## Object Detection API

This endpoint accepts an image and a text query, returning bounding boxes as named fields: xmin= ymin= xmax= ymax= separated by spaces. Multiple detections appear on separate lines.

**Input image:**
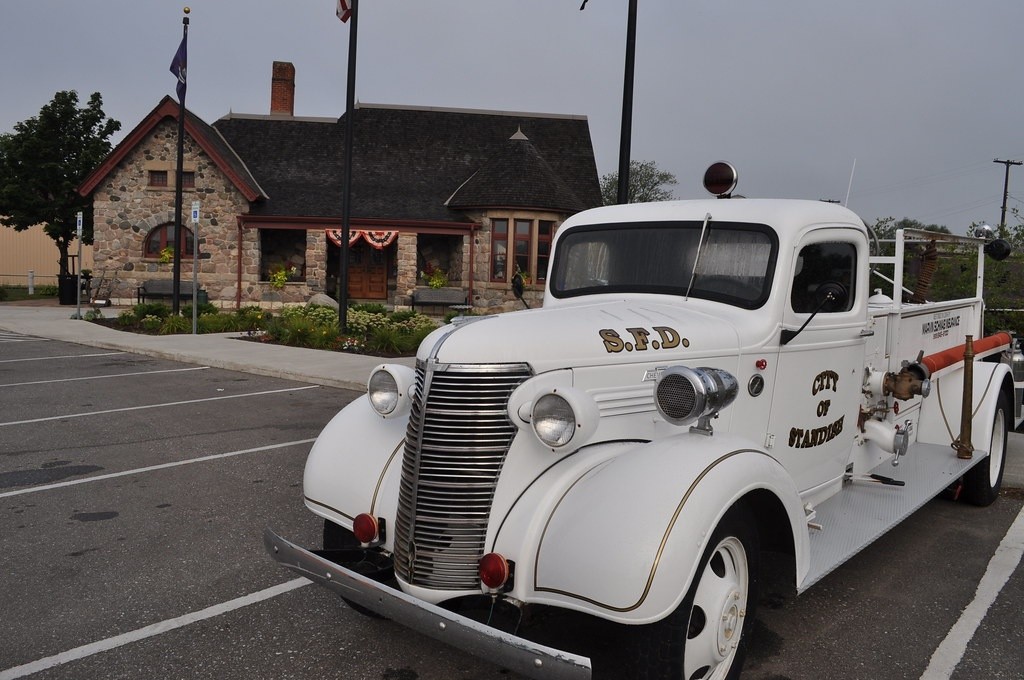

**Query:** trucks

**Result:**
xmin=263 ymin=158 xmax=1024 ymax=679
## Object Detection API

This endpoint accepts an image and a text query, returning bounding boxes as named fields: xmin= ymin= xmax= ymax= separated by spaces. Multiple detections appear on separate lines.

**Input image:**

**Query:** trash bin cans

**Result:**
xmin=56 ymin=274 xmax=79 ymax=305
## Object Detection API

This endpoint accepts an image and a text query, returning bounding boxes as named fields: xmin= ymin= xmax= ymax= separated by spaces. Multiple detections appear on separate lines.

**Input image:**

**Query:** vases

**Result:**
xmin=424 ymin=279 xmax=430 ymax=286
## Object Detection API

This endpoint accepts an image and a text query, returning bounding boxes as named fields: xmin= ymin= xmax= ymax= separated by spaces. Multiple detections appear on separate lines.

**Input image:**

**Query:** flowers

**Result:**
xmin=420 ymin=258 xmax=449 ymax=289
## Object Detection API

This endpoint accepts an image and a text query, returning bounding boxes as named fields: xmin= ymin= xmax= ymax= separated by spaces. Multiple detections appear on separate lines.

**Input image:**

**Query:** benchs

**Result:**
xmin=137 ymin=280 xmax=199 ymax=304
xmin=409 ymin=288 xmax=468 ymax=311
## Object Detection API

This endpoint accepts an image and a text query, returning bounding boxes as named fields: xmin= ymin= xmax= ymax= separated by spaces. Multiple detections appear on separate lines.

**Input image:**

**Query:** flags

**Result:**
xmin=169 ymin=26 xmax=187 ymax=107
xmin=336 ymin=0 xmax=351 ymax=23
